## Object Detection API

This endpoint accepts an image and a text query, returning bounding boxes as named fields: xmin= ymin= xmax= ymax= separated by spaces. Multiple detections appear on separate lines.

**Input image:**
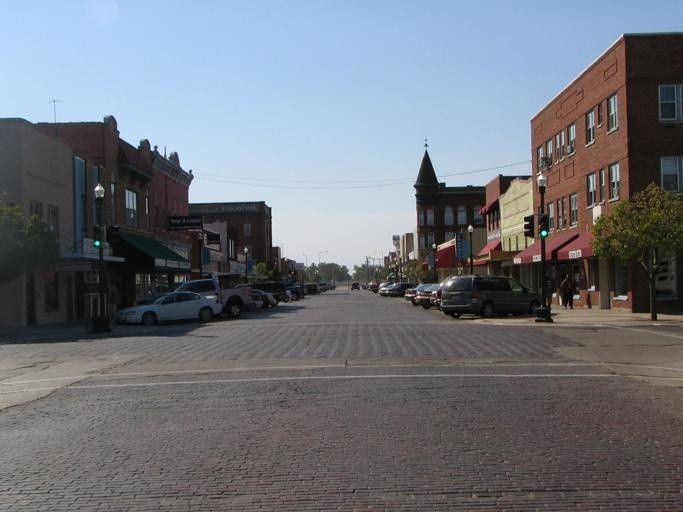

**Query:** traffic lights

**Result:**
xmin=93 ymin=225 xmax=120 ymax=249
xmin=524 ymin=214 xmax=549 ymax=238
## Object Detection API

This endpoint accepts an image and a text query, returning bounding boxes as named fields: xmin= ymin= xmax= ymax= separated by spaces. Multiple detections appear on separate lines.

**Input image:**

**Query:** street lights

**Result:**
xmin=292 ymin=272 xmax=293 ymax=276
xmin=198 ymin=233 xmax=202 ymax=279
xmin=376 ymin=268 xmax=380 ymax=281
xmin=244 ymin=247 xmax=248 ymax=282
xmin=535 ymin=174 xmax=553 ymax=321
xmin=389 ymin=255 xmax=402 ymax=284
xmin=94 ymin=184 xmax=105 ymax=317
xmin=284 ymin=257 xmax=288 ymax=280
xmin=468 ymin=224 xmax=473 ymax=275
xmin=433 ymin=243 xmax=436 ymax=283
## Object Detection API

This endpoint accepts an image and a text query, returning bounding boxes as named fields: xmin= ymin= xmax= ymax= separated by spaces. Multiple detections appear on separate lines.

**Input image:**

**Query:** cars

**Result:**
xmin=118 ymin=278 xmax=336 ymax=325
xmin=363 ymin=275 xmax=541 ymax=318
xmin=352 ymin=283 xmax=360 ymax=290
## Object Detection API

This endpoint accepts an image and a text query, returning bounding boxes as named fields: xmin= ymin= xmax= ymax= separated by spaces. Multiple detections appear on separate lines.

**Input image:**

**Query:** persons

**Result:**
xmin=540 ymin=272 xmax=554 ymax=307
xmin=559 ymin=272 xmax=576 ymax=308
